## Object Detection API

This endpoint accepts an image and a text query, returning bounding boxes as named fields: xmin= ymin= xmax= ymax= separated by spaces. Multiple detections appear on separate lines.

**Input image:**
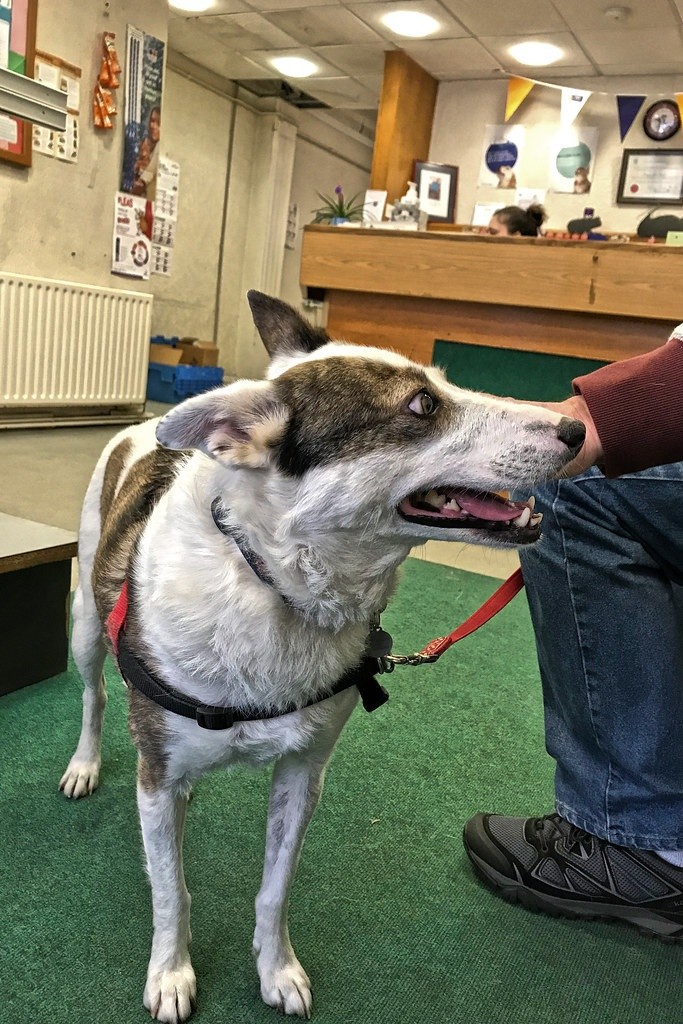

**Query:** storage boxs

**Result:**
xmin=0 ymin=270 xmax=153 ymax=410
xmin=175 ymin=336 xmax=219 ymax=367
xmin=148 ymin=363 xmax=224 ymax=404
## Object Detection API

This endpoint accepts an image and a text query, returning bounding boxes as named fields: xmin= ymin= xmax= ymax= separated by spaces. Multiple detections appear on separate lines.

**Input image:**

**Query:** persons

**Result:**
xmin=486 ymin=202 xmax=546 ymax=239
xmin=132 ymin=107 xmax=160 ymax=201
xmin=460 ymin=311 xmax=683 ymax=949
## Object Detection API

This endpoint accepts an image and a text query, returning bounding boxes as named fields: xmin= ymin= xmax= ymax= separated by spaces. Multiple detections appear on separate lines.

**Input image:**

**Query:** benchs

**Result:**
xmin=0 ymin=510 xmax=78 ymax=696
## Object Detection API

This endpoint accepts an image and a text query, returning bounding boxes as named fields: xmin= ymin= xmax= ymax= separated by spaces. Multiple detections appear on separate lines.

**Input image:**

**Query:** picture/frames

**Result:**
xmin=410 ymin=158 xmax=458 ymax=224
xmin=616 ymin=148 xmax=683 ymax=206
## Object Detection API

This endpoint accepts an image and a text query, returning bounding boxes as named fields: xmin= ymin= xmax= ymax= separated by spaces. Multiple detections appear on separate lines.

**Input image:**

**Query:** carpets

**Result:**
xmin=0 ymin=555 xmax=683 ymax=1024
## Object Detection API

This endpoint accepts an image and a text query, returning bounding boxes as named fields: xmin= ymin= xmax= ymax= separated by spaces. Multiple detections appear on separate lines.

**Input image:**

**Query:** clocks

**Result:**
xmin=642 ymin=99 xmax=681 ymax=141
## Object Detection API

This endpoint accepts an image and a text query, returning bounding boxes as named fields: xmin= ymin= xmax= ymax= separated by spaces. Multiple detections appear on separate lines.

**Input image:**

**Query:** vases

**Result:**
xmin=329 ymin=217 xmax=350 ymax=226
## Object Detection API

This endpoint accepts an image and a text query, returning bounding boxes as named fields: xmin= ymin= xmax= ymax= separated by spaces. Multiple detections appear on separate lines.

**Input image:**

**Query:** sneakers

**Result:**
xmin=463 ymin=812 xmax=683 ymax=941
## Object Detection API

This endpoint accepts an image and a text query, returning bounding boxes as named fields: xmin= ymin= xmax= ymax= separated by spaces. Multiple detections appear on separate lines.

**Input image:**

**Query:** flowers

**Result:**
xmin=299 ymin=185 xmax=377 ymax=230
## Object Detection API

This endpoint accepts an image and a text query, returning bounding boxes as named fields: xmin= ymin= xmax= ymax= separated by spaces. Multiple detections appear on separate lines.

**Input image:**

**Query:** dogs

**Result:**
xmin=57 ymin=287 xmax=589 ymax=1023
